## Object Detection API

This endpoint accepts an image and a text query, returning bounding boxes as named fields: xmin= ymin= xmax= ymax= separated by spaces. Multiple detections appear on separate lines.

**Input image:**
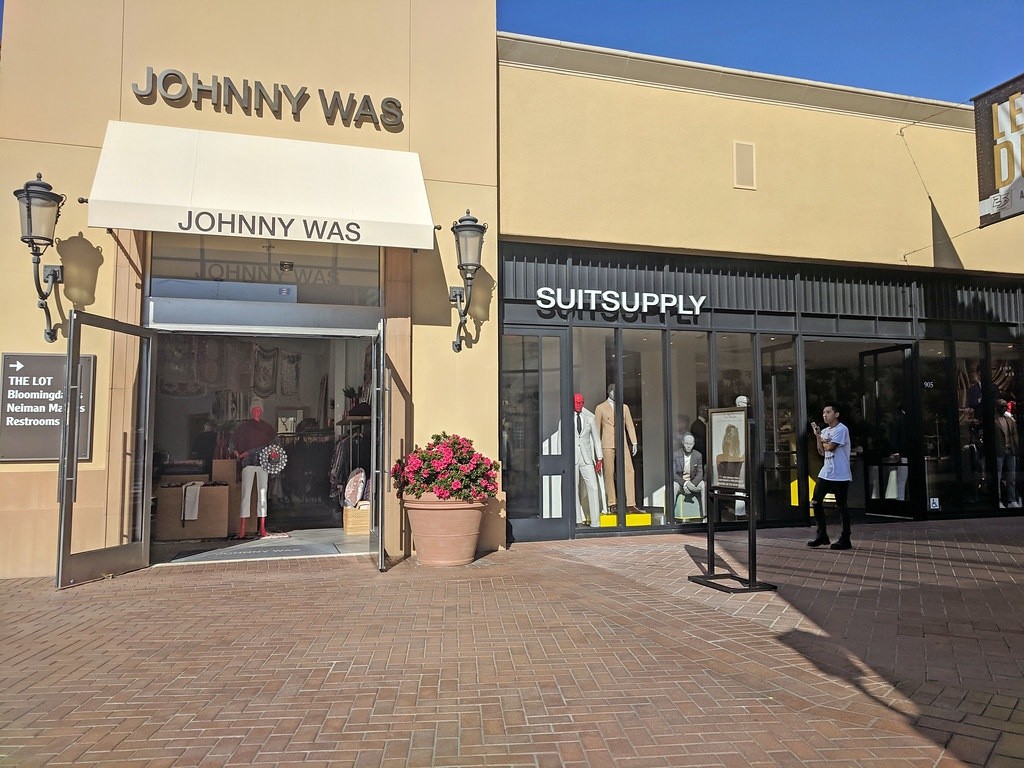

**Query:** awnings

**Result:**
xmin=86 ymin=119 xmax=434 ymax=250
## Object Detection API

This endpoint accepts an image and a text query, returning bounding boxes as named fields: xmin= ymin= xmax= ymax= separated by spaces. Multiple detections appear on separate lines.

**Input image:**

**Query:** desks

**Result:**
xmin=154 ymin=459 xmax=257 ymax=538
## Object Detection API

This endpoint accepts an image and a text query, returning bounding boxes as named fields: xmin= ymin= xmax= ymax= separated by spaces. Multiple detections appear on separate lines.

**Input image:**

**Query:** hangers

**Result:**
xmin=277 ymin=428 xmax=364 ymax=446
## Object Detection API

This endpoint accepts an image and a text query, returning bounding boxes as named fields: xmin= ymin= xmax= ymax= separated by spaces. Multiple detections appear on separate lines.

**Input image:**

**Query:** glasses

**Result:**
xmin=1000 ymin=405 xmax=1008 ymax=409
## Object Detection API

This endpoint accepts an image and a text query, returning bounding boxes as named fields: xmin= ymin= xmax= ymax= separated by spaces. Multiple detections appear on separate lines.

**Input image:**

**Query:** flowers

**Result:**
xmin=389 ymin=431 xmax=500 ymax=503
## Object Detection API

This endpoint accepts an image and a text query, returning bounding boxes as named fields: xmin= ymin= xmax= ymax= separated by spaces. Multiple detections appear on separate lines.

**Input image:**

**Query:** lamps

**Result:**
xmin=13 ymin=173 xmax=63 ymax=342
xmin=449 ymin=207 xmax=488 ymax=351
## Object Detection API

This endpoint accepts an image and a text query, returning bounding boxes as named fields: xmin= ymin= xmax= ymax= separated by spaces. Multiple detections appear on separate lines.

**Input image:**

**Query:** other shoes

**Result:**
xmin=703 ymin=518 xmax=707 ymax=523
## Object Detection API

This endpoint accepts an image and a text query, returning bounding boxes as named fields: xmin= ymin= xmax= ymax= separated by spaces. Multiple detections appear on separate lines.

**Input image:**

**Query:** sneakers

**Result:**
xmin=999 ymin=503 xmax=1006 ymax=508
xmin=807 ymin=533 xmax=830 ymax=547
xmin=1007 ymin=501 xmax=1022 ymax=508
xmin=830 ymin=538 xmax=852 ymax=549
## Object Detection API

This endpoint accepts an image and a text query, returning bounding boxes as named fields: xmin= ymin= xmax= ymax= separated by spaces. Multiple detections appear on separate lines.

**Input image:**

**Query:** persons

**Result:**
xmin=994 ymin=399 xmax=1020 ymax=508
xmin=191 ymin=418 xmax=218 ymax=462
xmin=594 ymin=383 xmax=646 ymax=513
xmin=228 ymin=396 xmax=276 ymax=537
xmin=671 ymin=431 xmax=708 ymax=523
xmin=556 ymin=392 xmax=604 ymax=527
xmin=805 ymin=405 xmax=853 ymax=550
xmin=735 ymin=395 xmax=750 ymax=409
xmin=690 ymin=404 xmax=709 ymax=476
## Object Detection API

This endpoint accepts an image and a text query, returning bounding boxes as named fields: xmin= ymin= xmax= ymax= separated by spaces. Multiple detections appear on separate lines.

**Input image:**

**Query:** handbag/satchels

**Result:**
xmin=278 ymin=417 xmax=296 ymax=433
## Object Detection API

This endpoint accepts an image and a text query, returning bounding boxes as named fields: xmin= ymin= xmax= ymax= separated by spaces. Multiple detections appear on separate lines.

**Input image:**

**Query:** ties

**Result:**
xmin=576 ymin=413 xmax=582 ymax=435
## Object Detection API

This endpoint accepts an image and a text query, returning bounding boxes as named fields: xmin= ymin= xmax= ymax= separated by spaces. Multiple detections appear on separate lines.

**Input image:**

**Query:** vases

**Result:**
xmin=401 ymin=491 xmax=488 ymax=566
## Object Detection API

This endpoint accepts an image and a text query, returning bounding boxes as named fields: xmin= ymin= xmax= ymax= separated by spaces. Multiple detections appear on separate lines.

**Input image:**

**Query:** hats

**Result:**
xmin=250 ymin=396 xmax=263 ymax=413
xmin=608 ymin=384 xmax=615 ymax=394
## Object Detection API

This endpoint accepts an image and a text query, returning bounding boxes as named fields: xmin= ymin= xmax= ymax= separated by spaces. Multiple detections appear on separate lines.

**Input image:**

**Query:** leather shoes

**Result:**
xmin=627 ymin=506 xmax=646 ymax=514
xmin=611 ymin=505 xmax=618 ymax=512
